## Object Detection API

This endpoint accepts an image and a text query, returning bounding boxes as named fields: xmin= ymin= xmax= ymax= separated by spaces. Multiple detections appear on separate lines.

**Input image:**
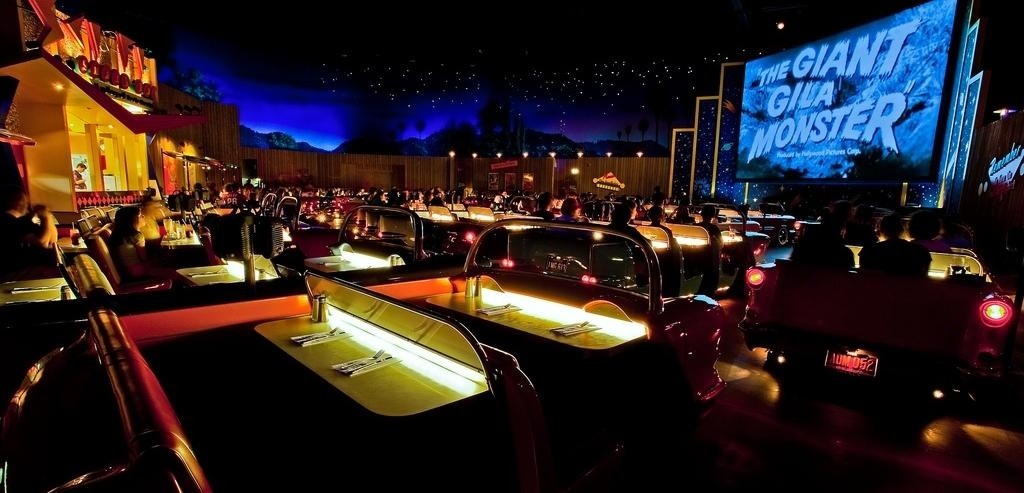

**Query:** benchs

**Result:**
xmin=1 ymin=184 xmax=1023 ymax=490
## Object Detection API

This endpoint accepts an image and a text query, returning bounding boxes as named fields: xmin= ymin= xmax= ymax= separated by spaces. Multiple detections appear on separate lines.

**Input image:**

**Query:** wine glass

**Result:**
xmin=163 ymin=219 xmax=181 ymax=239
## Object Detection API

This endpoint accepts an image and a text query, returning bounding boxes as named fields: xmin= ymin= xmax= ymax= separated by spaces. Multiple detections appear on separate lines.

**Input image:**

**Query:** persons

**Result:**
xmin=73 ymin=163 xmax=87 ymax=189
xmin=1 ymin=172 xmax=58 ymax=281
xmin=108 ymin=179 xmax=953 ymax=280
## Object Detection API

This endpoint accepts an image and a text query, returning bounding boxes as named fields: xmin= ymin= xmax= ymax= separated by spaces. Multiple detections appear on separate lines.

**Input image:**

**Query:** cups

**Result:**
xmin=175 ymin=225 xmax=184 ymax=240
xmin=70 ymin=229 xmax=80 ymax=246
xmin=185 ymin=224 xmax=194 ymax=239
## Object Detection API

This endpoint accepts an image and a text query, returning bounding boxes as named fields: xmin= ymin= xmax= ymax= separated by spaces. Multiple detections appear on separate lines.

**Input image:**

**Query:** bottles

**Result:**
xmin=474 ymin=274 xmax=482 ymax=297
xmin=312 ymin=294 xmax=328 ymax=323
xmin=464 ymin=275 xmax=473 ymax=299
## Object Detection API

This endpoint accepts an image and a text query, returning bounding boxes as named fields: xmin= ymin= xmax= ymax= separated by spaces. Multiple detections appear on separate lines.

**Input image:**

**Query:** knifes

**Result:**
xmin=343 ymin=356 xmax=395 ymax=379
xmin=297 ymin=331 xmax=346 ymax=346
xmin=559 ymin=327 xmax=604 ymax=337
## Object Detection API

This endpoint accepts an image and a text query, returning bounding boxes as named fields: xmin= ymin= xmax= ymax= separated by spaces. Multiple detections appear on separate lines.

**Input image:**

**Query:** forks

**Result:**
xmin=552 ymin=320 xmax=589 ymax=333
xmin=477 ymin=303 xmax=511 ymax=314
xmin=292 ymin=326 xmax=341 ymax=342
xmin=13 ymin=281 xmax=63 ymax=290
xmin=340 ymin=348 xmax=385 ymax=370
